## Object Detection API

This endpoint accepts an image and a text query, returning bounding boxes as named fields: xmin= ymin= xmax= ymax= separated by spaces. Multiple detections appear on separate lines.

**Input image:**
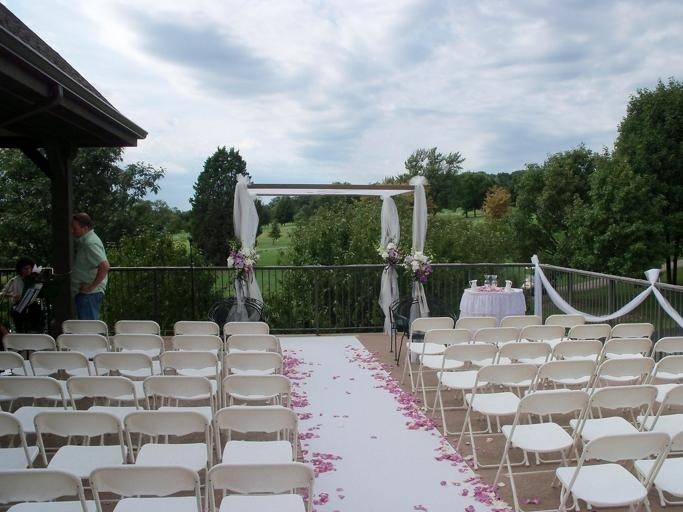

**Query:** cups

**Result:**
xmin=482 ymin=274 xmax=497 ymax=289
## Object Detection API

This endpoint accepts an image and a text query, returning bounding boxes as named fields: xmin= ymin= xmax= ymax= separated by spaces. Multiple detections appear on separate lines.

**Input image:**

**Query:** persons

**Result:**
xmin=66 ymin=211 xmax=112 ymax=320
xmin=0 ymin=256 xmax=47 ymax=334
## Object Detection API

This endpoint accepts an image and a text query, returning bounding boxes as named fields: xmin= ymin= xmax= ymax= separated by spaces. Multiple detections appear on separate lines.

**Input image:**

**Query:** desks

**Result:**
xmin=458 ymin=286 xmax=526 ymax=325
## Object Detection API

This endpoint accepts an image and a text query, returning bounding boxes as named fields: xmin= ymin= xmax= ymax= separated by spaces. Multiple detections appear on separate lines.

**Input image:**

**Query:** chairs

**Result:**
xmin=140 ymin=376 xmax=215 ymax=453
xmin=90 ymin=464 xmax=203 ymax=512
xmin=158 ymin=351 xmax=219 ymax=408
xmin=406 ymin=316 xmax=454 ymax=396
xmin=174 ymin=320 xmax=220 ymax=336
xmin=62 ymin=319 xmax=108 ymax=335
xmin=0 ymin=351 xmax=32 ymax=411
xmin=222 ymin=352 xmax=283 ymax=405
xmin=555 ymin=431 xmax=670 ymax=510
xmin=429 ymin=343 xmax=494 ymax=436
xmin=454 ymin=314 xmax=682 ymax=365
xmin=28 ymin=351 xmax=92 ymax=408
xmin=66 ymin=375 xmax=143 ymax=449
xmin=93 ymin=352 xmax=155 ymax=408
xmin=413 ymin=329 xmax=470 ymax=412
xmin=223 ymin=322 xmax=270 ymax=334
xmin=171 ymin=335 xmax=224 ymax=376
xmin=634 ymin=431 xmax=681 ymax=510
xmin=489 ymin=387 xmax=590 ymax=512
xmin=521 ymin=359 xmax=596 ymax=465
xmin=34 ymin=409 xmax=127 ymax=503
xmin=56 ymin=334 xmax=111 ymax=376
xmin=112 ymin=333 xmax=164 ymax=373
xmin=585 ymin=357 xmax=654 ymax=423
xmin=0 ymin=410 xmax=38 ymax=468
xmin=637 ymin=383 xmax=683 ymax=451
xmin=0 ymin=374 xmax=75 ymax=448
xmin=216 ymin=405 xmax=299 ymax=497
xmin=221 ymin=374 xmax=291 ymax=441
xmin=457 ymin=363 xmax=538 ymax=471
xmin=651 ymin=354 xmax=681 ymax=385
xmin=123 ymin=410 xmax=213 ymax=511
xmin=226 ymin=334 xmax=283 ymax=376
xmin=114 ymin=319 xmax=161 ymax=335
xmin=206 ymin=463 xmax=312 ymax=512
xmin=0 ymin=467 xmax=89 ymax=512
xmin=3 ymin=333 xmax=56 ymax=374
xmin=556 ymin=384 xmax=659 ymax=488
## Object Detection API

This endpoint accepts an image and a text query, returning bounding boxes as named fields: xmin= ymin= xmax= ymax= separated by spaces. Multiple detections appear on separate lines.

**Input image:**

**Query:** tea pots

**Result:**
xmin=505 ymin=280 xmax=512 ymax=291
xmin=468 ymin=279 xmax=478 ymax=292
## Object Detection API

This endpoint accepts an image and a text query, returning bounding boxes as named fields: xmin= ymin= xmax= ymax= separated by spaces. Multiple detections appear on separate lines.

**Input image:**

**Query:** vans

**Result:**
xmin=520 ymin=275 xmax=556 ymax=295
